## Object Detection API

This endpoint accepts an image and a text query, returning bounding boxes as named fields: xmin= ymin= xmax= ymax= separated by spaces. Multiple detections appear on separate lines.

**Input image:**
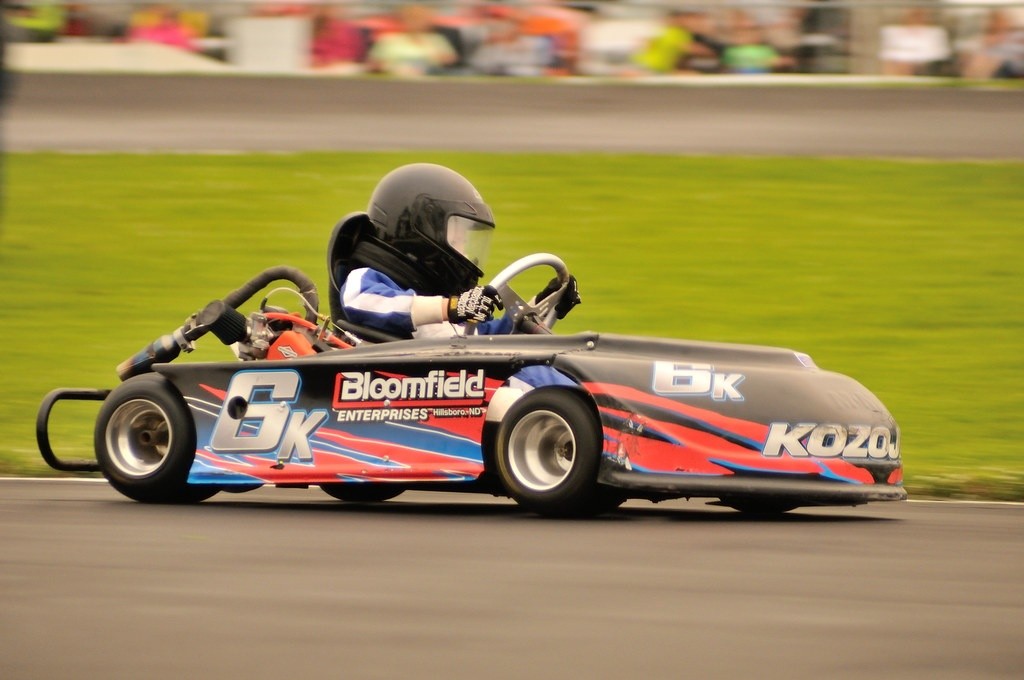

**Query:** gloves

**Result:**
xmin=447 ymin=285 xmax=503 ymax=324
xmin=535 ymin=274 xmax=581 ymax=320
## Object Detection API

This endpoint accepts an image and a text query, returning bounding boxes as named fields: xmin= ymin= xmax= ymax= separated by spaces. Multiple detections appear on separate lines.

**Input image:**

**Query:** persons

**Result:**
xmin=340 ymin=163 xmax=584 ymax=342
xmin=3 ymin=1 xmax=1024 ymax=83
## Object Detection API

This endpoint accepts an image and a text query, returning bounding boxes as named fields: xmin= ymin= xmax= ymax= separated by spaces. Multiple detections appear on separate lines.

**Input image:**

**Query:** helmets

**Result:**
xmin=367 ymin=163 xmax=496 ymax=298
